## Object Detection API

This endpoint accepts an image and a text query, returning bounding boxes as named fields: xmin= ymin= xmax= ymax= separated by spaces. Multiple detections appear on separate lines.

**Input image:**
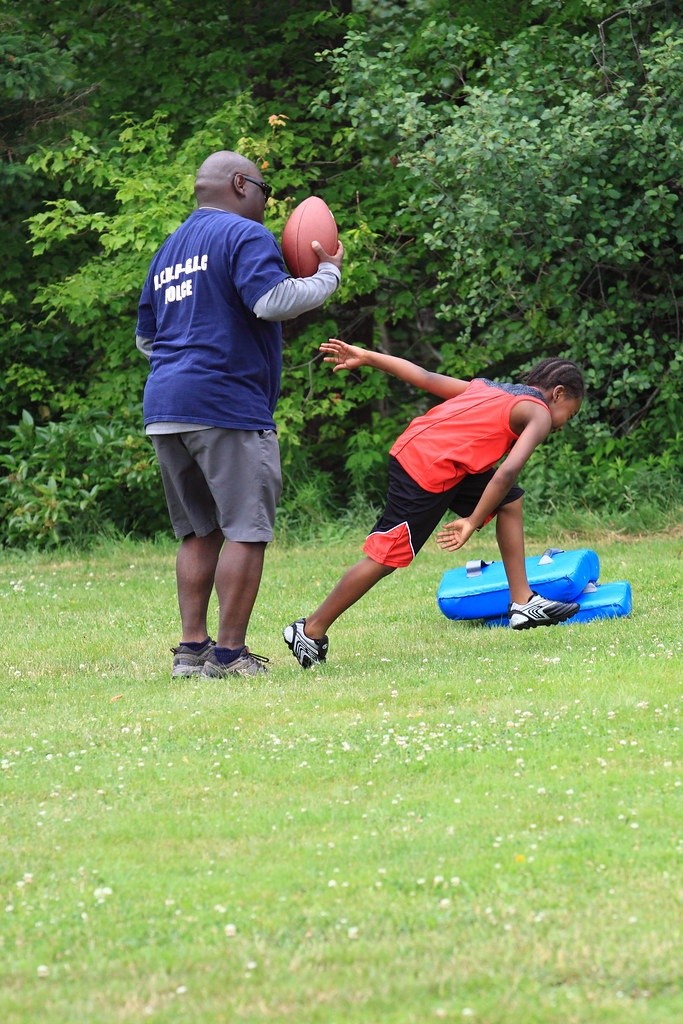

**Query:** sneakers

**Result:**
xmin=283 ymin=618 xmax=329 ymax=670
xmin=508 ymin=590 xmax=580 ymax=630
xmin=170 ymin=637 xmax=216 ymax=679
xmin=201 ymin=646 xmax=269 ymax=681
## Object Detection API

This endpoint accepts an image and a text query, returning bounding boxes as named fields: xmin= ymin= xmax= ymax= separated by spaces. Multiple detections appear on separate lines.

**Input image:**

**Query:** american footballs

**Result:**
xmin=282 ymin=196 xmax=338 ymax=279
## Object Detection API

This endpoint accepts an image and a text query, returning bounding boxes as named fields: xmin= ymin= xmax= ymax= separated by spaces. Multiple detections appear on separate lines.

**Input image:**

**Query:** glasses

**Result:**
xmin=235 ymin=173 xmax=272 ymax=204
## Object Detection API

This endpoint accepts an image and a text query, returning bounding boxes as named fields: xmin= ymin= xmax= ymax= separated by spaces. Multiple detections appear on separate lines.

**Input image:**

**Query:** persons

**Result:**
xmin=281 ymin=336 xmax=583 ymax=668
xmin=133 ymin=149 xmax=344 ymax=685
